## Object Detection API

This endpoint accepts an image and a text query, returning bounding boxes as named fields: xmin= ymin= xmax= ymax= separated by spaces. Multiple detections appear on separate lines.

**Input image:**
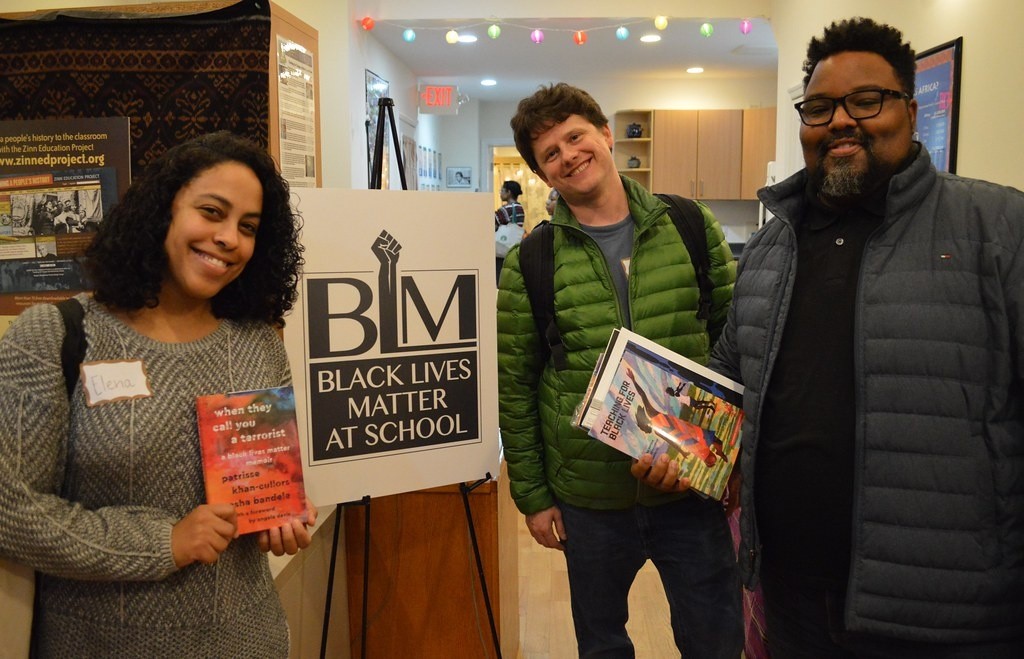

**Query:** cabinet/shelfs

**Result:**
xmin=612 ymin=109 xmax=777 ymax=200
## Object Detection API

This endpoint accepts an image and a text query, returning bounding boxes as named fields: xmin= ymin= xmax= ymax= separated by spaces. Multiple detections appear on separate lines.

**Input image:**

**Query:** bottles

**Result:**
xmin=627 ymin=156 xmax=640 ymax=168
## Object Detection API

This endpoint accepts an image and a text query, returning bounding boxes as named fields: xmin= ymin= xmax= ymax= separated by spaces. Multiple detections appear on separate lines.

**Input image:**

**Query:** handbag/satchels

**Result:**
xmin=495 ymin=202 xmax=527 ymax=259
xmin=0 ymin=298 xmax=89 ymax=659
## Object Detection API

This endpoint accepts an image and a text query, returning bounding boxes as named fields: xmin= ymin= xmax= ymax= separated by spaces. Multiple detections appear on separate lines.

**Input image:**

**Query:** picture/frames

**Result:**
xmin=365 ymin=69 xmax=390 ymax=190
xmin=446 ymin=167 xmax=472 ymax=188
xmin=915 ymin=36 xmax=964 ymax=177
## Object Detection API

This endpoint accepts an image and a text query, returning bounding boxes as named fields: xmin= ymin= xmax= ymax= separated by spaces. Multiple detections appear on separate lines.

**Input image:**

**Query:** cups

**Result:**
xmin=627 ymin=124 xmax=643 ymax=138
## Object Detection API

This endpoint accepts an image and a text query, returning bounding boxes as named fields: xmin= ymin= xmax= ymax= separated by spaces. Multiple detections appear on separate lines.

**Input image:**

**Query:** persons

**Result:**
xmin=452 ymin=172 xmax=470 ymax=185
xmin=630 ymin=16 xmax=1024 ymax=659
xmin=545 ymin=187 xmax=560 ymax=215
xmin=0 ymin=129 xmax=318 ymax=659
xmin=497 ymin=84 xmax=744 ymax=659
xmin=495 ymin=180 xmax=525 ymax=289
xmin=34 ymin=193 xmax=88 ymax=235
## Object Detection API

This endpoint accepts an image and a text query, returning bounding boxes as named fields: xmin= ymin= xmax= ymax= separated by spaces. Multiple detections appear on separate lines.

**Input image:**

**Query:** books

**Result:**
xmin=194 ymin=385 xmax=309 ymax=540
xmin=571 ymin=324 xmax=746 ymax=500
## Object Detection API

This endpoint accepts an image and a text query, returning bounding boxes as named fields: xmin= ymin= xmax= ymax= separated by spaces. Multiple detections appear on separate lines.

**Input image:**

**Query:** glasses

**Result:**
xmin=794 ymin=86 xmax=910 ymax=127
